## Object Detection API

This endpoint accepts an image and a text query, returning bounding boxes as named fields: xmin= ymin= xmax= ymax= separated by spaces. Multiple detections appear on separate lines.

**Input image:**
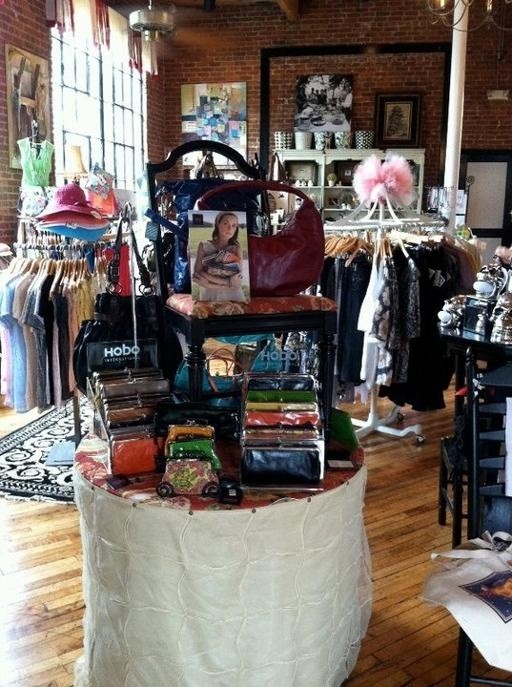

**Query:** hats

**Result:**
xmin=33 ymin=174 xmax=112 ymax=242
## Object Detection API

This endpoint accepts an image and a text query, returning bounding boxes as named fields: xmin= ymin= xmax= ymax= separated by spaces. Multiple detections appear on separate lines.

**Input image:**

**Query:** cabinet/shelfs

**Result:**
xmin=275 ymin=146 xmax=423 ymax=224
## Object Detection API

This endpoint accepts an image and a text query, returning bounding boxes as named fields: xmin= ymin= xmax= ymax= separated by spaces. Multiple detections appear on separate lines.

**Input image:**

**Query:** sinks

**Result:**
xmin=449 ymin=295 xmax=499 ymax=335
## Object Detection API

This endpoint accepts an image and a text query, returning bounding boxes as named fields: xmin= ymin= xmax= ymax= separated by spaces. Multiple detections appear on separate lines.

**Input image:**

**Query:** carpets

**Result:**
xmin=0 ymin=395 xmax=90 ymax=502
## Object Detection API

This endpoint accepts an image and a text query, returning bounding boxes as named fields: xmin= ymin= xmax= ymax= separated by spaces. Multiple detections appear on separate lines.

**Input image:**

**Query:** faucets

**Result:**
xmin=480 ymin=263 xmax=509 ymax=301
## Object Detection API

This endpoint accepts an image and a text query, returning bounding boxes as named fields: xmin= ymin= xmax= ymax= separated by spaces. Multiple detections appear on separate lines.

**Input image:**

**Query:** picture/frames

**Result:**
xmin=373 ymin=89 xmax=424 ymax=147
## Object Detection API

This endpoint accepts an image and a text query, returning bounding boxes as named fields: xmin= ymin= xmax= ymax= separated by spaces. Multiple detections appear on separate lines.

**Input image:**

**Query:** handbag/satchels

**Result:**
xmin=415 ymin=529 xmax=512 ymax=672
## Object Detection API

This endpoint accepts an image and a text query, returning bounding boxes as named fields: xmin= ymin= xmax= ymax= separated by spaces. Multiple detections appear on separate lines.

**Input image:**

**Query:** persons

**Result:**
xmin=307 ymin=87 xmax=327 ymax=106
xmin=192 ymin=211 xmax=248 ymax=300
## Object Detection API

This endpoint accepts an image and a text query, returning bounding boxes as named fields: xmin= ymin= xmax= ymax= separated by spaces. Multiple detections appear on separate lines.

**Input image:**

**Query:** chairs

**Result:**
xmin=436 ymin=342 xmax=512 ymax=687
xmin=145 ymin=137 xmax=338 ymax=430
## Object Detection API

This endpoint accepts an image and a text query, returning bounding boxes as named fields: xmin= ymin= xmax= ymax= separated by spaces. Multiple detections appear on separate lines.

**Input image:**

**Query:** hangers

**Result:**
xmin=323 ymin=231 xmax=484 ymax=269
xmin=5 ymin=242 xmax=110 ymax=280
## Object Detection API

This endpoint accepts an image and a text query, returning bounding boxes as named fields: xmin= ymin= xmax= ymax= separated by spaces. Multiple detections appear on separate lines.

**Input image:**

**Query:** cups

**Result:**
xmin=274 ymin=129 xmax=377 ymax=150
